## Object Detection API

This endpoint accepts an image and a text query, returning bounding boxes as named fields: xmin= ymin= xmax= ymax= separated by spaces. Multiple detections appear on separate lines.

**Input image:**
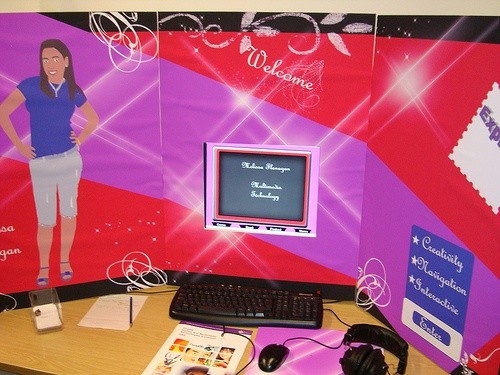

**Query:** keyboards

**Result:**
xmin=169 ymin=284 xmax=322 ymax=329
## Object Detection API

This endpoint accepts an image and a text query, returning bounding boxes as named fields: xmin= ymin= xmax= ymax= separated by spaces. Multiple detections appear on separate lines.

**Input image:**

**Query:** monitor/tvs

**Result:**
xmin=202 ymin=142 xmax=320 ymax=238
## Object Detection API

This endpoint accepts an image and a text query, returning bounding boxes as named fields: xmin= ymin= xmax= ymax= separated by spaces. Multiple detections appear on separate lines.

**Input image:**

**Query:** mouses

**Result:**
xmin=258 ymin=344 xmax=289 ymax=373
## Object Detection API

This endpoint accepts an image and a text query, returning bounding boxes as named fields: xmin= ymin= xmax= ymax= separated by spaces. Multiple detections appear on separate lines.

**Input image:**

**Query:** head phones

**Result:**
xmin=340 ymin=324 xmax=409 ymax=375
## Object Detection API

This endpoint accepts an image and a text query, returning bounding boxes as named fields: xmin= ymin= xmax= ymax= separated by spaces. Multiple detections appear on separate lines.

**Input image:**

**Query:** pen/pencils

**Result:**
xmin=130 ymin=296 xmax=132 ymax=327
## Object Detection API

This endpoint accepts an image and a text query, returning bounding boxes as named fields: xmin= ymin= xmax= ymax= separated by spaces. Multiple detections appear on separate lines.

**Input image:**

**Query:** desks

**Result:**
xmin=0 ymin=284 xmax=453 ymax=375
